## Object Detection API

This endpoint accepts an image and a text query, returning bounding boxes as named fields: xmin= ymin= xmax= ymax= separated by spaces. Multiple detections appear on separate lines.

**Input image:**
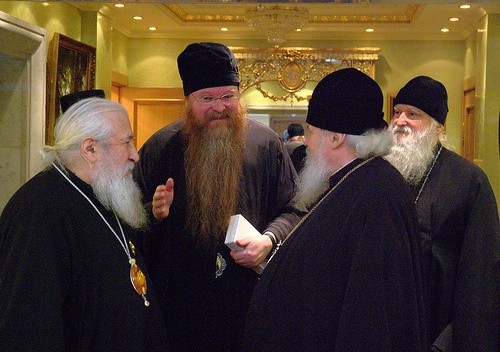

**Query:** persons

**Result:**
xmin=132 ymin=42 xmax=302 ymax=352
xmin=0 ymin=98 xmax=167 ymax=352
xmin=241 ymin=68 xmax=428 ymax=352
xmin=382 ymin=76 xmax=500 ymax=352
xmin=286 ymin=124 xmax=307 ymax=175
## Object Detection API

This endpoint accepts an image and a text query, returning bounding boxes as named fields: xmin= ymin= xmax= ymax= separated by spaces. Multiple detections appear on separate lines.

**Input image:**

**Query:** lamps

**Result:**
xmin=244 ymin=2 xmax=310 ymax=49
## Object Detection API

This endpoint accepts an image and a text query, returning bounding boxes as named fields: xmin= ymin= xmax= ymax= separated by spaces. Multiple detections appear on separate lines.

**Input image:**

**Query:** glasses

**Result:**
xmin=191 ymin=93 xmax=239 ymax=105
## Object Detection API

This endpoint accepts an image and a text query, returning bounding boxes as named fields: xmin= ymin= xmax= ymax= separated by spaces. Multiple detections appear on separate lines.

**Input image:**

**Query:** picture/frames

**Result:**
xmin=386 ymin=91 xmax=397 ymax=124
xmin=45 ymin=31 xmax=97 ymax=148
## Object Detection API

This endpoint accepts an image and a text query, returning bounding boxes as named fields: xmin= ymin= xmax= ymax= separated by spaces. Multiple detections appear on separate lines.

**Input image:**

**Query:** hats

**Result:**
xmin=177 ymin=41 xmax=240 ymax=97
xmin=394 ymin=75 xmax=449 ymax=126
xmin=287 ymin=124 xmax=304 ymax=139
xmin=306 ymin=67 xmax=389 ymax=136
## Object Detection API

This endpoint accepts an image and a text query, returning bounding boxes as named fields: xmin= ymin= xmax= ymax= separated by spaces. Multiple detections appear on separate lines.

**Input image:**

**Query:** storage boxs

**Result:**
xmin=224 ymin=214 xmax=269 ymax=277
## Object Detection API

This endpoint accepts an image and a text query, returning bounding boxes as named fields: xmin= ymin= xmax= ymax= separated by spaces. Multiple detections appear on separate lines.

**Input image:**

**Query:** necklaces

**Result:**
xmin=267 ymin=155 xmax=377 ymax=264
xmin=415 ymin=145 xmax=443 ymax=202
xmin=51 ymin=160 xmax=149 ymax=306
